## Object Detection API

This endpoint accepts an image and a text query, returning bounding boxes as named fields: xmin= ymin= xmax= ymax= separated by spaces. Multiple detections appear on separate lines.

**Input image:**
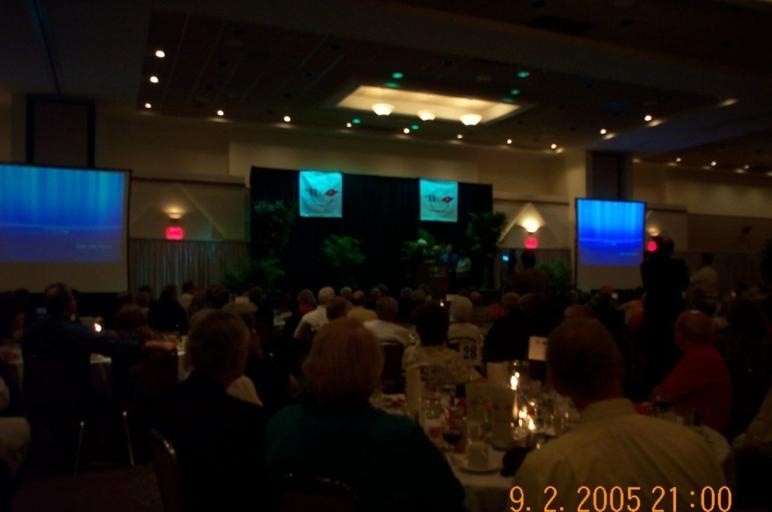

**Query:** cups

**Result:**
xmin=399 ymin=361 xmax=577 ymax=462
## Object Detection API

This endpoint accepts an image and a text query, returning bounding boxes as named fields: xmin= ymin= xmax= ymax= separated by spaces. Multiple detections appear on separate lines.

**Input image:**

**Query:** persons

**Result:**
xmin=1 ymin=235 xmax=772 ymax=510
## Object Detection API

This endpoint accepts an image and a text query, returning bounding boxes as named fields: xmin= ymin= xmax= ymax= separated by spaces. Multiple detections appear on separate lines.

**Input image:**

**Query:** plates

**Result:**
xmin=460 ymin=460 xmax=499 ymax=475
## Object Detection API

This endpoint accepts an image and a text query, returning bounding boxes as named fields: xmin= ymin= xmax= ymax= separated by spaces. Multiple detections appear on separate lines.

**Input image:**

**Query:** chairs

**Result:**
xmin=377 ymin=338 xmax=405 ymax=392
xmin=447 ymin=336 xmax=477 ymax=356
